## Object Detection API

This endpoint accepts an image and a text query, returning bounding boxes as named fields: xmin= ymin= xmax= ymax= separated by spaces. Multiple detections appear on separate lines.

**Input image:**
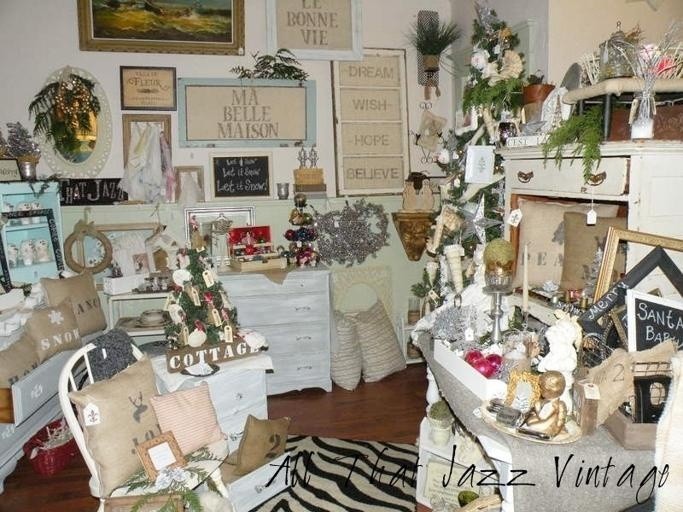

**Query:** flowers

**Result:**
xmin=463 ymin=2 xmax=525 ymax=110
xmin=7 ymin=122 xmax=41 ymax=162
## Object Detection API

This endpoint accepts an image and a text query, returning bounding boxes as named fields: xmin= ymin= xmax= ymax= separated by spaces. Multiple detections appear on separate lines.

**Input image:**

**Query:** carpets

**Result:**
xmin=249 ymin=433 xmax=420 ymax=512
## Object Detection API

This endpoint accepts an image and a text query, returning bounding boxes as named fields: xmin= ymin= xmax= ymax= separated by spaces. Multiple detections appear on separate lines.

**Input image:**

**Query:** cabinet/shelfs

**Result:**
xmin=214 ymin=259 xmax=335 ymax=396
xmin=492 ymin=137 xmax=682 ymax=333
xmin=0 ymin=329 xmax=106 ymax=494
xmin=150 ymin=353 xmax=274 ymax=435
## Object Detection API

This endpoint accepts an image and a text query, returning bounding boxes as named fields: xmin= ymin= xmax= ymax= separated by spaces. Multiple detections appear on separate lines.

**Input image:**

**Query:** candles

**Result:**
xmin=522 ymin=244 xmax=528 ymax=311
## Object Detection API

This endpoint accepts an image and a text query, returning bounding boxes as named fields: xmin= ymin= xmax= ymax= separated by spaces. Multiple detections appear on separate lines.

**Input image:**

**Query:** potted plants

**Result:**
xmin=406 ymin=335 xmax=422 ymax=359
xmin=523 ymin=74 xmax=556 ymax=102
xmin=483 ymin=238 xmax=515 ymax=291
xmin=402 ymin=19 xmax=462 ymax=70
xmin=406 ymin=297 xmax=419 ymax=324
xmin=425 ymin=401 xmax=454 ymax=448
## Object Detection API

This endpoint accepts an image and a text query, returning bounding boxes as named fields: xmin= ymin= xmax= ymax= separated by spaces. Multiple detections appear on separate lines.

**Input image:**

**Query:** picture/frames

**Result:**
xmin=119 ymin=66 xmax=177 ymax=113
xmin=266 ymin=1 xmax=363 ymax=63
xmin=0 ymin=209 xmax=65 ymax=291
xmin=135 ymin=430 xmax=184 ymax=481
xmin=184 ymin=205 xmax=257 ymax=269
xmin=79 ymin=224 xmax=165 ymax=287
xmin=0 ymin=181 xmax=65 ymax=283
xmin=170 ymin=164 xmax=205 ymax=202
xmin=76 ymin=0 xmax=247 ymax=58
xmin=330 ymin=45 xmax=410 ymax=196
xmin=575 ymin=245 xmax=682 ymax=348
xmin=0 ymin=158 xmax=22 ymax=180
xmin=175 ymin=76 xmax=316 ymax=149
xmin=35 ymin=64 xmax=112 ymax=180
xmin=121 ymin=114 xmax=171 ymax=170
xmin=594 ymin=226 xmax=683 ymax=306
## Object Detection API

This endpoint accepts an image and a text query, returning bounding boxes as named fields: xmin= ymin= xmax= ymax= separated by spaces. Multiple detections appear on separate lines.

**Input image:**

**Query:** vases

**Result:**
xmin=629 ymin=89 xmax=655 ymax=140
xmin=475 ymin=99 xmax=510 ymax=145
xmin=16 ymin=160 xmax=36 ymax=180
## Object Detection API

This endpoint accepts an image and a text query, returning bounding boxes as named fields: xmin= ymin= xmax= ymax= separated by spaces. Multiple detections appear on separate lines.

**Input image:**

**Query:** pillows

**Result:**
xmin=233 ymin=414 xmax=291 ymax=477
xmin=66 ymin=351 xmax=163 ymax=495
xmin=103 ymin=493 xmax=184 ymax=512
xmin=28 ymin=296 xmax=82 ymax=358
xmin=329 ymin=309 xmax=361 ymax=392
xmin=351 ymin=301 xmax=407 ymax=383
xmin=146 ymin=380 xmax=227 ymax=456
xmin=39 ymin=275 xmax=107 ymax=336
xmin=0 ymin=333 xmax=40 ymax=391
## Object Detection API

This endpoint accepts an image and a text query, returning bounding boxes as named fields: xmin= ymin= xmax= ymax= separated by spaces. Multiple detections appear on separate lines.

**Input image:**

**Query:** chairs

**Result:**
xmin=59 ymin=333 xmax=234 ymax=512
